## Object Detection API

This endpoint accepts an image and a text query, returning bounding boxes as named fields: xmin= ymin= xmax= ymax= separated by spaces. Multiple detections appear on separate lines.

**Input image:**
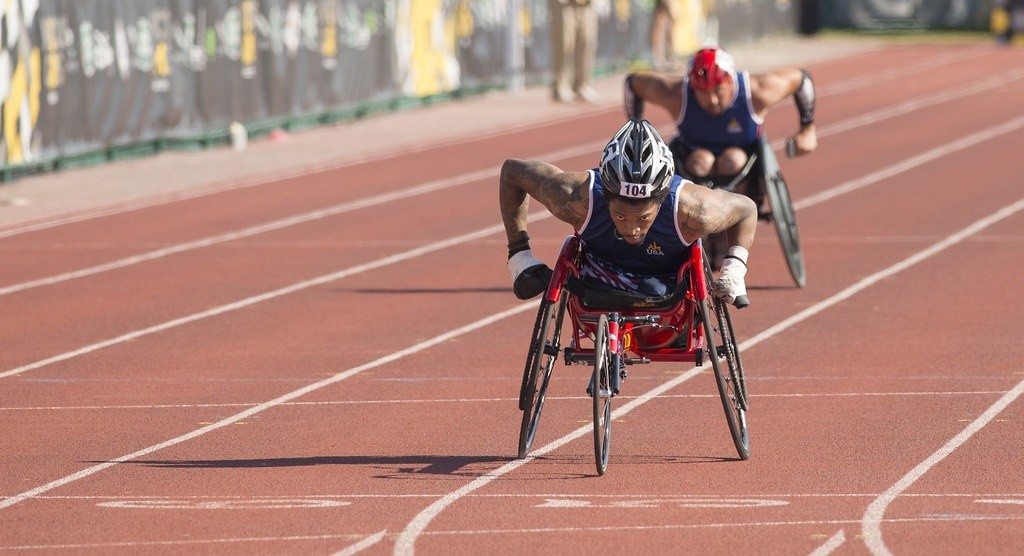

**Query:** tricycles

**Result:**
xmin=667 ymin=132 xmax=807 ymax=289
xmin=514 ymin=233 xmax=752 ymax=476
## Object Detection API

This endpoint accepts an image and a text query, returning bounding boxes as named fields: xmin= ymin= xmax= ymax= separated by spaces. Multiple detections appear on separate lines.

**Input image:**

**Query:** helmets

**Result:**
xmin=599 ymin=119 xmax=675 ymax=198
xmin=688 ymin=47 xmax=735 ymax=89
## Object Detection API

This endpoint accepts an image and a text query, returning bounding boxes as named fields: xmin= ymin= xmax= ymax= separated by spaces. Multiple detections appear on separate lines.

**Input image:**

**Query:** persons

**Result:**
xmin=546 ymin=0 xmax=600 ymax=110
xmin=622 ymin=46 xmax=819 ymax=271
xmin=649 ymin=0 xmax=682 ymax=70
xmin=500 ymin=119 xmax=758 ymax=351
